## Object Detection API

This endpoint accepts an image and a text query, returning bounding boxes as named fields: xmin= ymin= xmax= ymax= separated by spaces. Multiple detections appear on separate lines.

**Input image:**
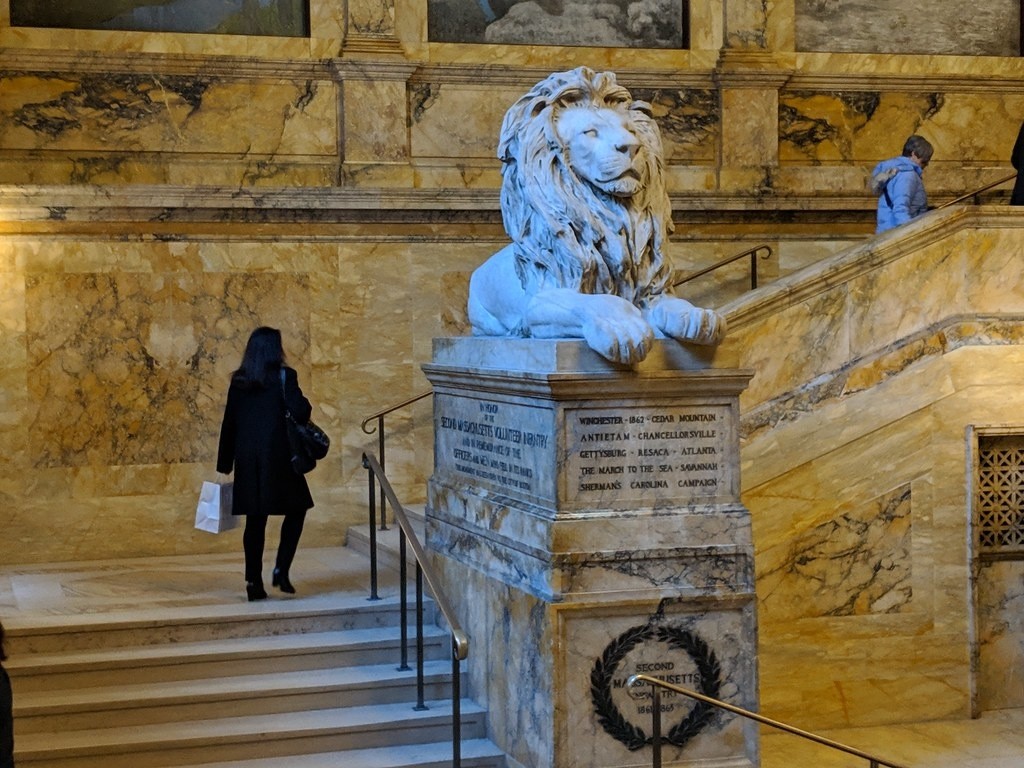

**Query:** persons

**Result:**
xmin=1011 ymin=121 xmax=1024 ymax=206
xmin=871 ymin=135 xmax=934 ymax=234
xmin=215 ymin=326 xmax=313 ymax=602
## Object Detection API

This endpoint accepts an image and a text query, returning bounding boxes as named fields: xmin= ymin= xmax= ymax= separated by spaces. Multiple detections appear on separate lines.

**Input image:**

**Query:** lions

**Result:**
xmin=466 ymin=68 xmax=730 ymax=365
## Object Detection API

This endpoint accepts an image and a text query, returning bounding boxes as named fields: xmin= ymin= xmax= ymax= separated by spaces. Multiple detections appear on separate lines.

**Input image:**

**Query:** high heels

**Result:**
xmin=246 ymin=580 xmax=267 ymax=602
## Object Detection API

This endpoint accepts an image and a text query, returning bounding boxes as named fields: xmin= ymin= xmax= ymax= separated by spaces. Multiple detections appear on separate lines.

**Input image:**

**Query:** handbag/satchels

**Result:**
xmin=280 ymin=366 xmax=330 ymax=475
xmin=194 ymin=472 xmax=241 ymax=534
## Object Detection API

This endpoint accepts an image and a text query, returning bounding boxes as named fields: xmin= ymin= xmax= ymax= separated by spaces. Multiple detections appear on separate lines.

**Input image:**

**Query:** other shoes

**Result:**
xmin=272 ymin=567 xmax=296 ymax=593
